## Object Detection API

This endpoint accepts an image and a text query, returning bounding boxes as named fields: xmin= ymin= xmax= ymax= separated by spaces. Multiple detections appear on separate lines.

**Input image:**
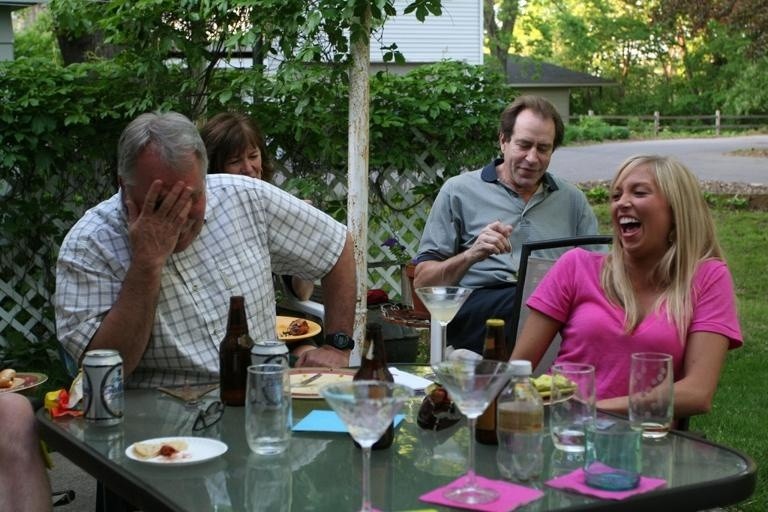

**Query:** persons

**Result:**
xmin=201 ymin=112 xmax=314 ymax=302
xmin=414 ymin=94 xmax=609 ymax=359
xmin=57 ymin=112 xmax=356 ymax=395
xmin=512 ymin=156 xmax=744 ymax=430
xmin=0 ymin=393 xmax=51 ymax=511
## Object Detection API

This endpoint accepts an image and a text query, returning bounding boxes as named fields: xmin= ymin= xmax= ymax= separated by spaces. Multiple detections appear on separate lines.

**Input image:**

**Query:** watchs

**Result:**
xmin=323 ymin=333 xmax=353 ymax=350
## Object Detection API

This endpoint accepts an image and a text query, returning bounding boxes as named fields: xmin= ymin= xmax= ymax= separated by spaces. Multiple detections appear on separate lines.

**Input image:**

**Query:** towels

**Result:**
xmin=419 ymin=470 xmax=546 ymax=512
xmin=292 ymin=409 xmax=406 ymax=433
xmin=545 ymin=457 xmax=668 ymax=500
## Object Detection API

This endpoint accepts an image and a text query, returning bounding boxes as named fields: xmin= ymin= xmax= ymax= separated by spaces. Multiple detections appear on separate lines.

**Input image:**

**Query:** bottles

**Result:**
xmin=475 ymin=320 xmax=514 ymax=446
xmin=354 ymin=289 xmax=394 ymax=450
xmin=250 ymin=340 xmax=291 ymax=366
xmin=220 ymin=296 xmax=254 ymax=407
xmin=496 ymin=360 xmax=543 ymax=482
xmin=82 ymin=350 xmax=125 ymax=430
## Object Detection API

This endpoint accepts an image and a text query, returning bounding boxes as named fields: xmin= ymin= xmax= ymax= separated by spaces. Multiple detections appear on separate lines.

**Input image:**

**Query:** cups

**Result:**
xmin=244 ymin=362 xmax=295 ymax=457
xmin=628 ymin=352 xmax=674 ymax=440
xmin=430 ymin=360 xmax=514 ymax=507
xmin=548 ymin=361 xmax=598 ymax=454
xmin=413 ymin=284 xmax=475 ymax=363
xmin=320 ymin=379 xmax=416 ymax=512
xmin=582 ymin=418 xmax=644 ymax=492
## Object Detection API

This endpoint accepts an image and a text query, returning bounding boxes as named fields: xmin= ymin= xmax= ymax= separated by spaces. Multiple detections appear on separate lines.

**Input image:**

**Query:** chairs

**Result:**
xmin=513 ymin=236 xmax=691 ymax=434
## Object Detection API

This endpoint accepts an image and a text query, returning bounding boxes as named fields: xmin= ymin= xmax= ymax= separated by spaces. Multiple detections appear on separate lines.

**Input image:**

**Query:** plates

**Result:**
xmin=125 ymin=435 xmax=228 ymax=468
xmin=276 ymin=315 xmax=323 ymax=342
xmin=287 ymin=365 xmax=358 ymax=401
xmin=0 ymin=371 xmax=50 ymax=395
